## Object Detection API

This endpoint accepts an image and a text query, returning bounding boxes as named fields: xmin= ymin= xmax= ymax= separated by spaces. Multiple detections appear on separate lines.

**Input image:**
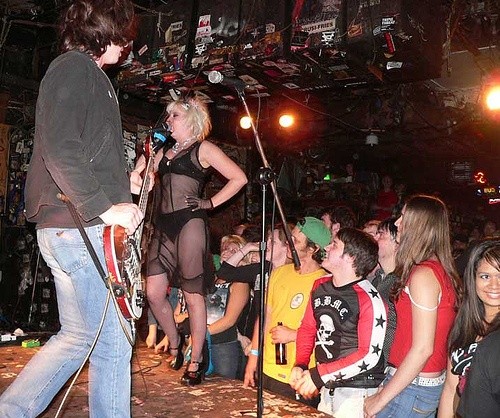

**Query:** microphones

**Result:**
xmin=256 ymin=166 xmax=274 ymax=185
xmin=208 ymin=70 xmax=250 ymax=89
xmin=148 ymin=128 xmax=172 ymax=158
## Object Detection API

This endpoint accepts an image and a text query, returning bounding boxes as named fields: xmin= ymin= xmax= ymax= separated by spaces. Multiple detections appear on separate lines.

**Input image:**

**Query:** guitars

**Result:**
xmin=103 ymin=132 xmax=155 ymax=321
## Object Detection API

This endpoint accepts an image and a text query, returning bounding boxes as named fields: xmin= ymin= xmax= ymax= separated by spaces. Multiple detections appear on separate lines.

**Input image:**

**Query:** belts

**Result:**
xmin=383 ymin=364 xmax=449 ymax=387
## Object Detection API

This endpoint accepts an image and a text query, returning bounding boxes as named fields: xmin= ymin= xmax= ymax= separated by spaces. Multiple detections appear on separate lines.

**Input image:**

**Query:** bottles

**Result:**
xmin=275 ymin=322 xmax=287 ymax=364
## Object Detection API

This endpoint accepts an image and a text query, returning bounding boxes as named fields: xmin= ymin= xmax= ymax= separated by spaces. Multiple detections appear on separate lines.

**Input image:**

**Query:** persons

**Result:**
xmin=438 ymin=237 xmax=500 ymax=418
xmin=364 ymin=195 xmax=462 ymax=418
xmin=145 ymin=162 xmax=500 ymax=409
xmin=134 ymin=94 xmax=248 ymax=386
xmin=0 ymin=0 xmax=154 ymax=418
xmin=456 ymin=331 xmax=499 ymax=418
xmin=290 ymin=228 xmax=387 ymax=418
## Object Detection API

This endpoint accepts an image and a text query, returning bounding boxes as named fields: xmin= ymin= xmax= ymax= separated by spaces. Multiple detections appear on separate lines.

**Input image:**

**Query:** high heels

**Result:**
xmin=169 ymin=332 xmax=185 ymax=370
xmin=179 ymin=358 xmax=207 ymax=385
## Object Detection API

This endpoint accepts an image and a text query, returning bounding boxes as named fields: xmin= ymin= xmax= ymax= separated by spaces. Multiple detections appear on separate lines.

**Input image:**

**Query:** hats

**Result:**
xmin=298 ymin=216 xmax=332 ymax=251
